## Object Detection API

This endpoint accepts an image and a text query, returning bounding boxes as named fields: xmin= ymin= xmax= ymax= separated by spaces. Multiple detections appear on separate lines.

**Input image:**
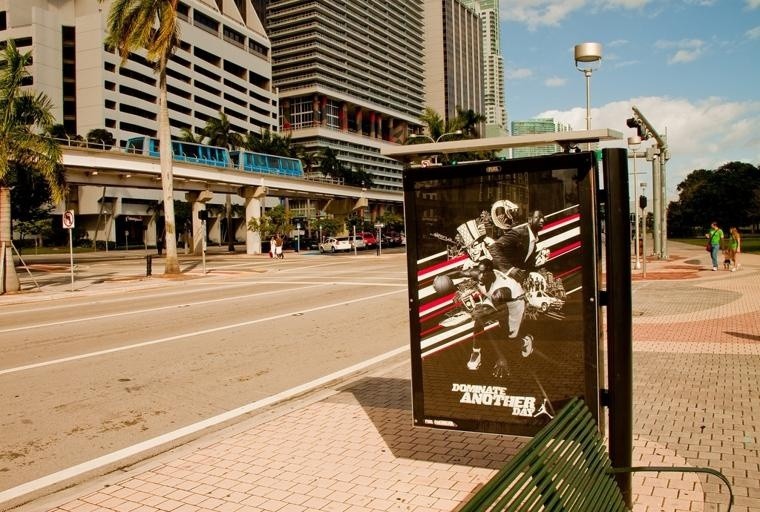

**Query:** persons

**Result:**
xmin=433 ymin=258 xmax=533 ymax=380
xmin=488 ymin=209 xmax=546 ymax=283
xmin=722 ymin=248 xmax=732 ymax=270
xmin=705 ymin=221 xmax=724 ymax=271
xmin=728 ymin=226 xmax=744 ymax=271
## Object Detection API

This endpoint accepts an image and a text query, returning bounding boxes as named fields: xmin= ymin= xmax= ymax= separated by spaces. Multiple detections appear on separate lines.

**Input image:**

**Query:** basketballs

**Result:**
xmin=433 ymin=275 xmax=453 ymax=294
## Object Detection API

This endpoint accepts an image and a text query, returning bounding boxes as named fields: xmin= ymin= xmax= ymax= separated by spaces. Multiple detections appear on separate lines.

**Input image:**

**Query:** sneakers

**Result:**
xmin=522 ymin=335 xmax=534 ymax=358
xmin=712 ymin=263 xmax=740 ymax=271
xmin=467 ymin=353 xmax=482 ymax=371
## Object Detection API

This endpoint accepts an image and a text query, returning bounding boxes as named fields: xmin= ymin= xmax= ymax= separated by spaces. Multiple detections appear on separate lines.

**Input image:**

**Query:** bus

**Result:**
xmin=229 ymin=149 xmax=304 ymax=179
xmin=125 ymin=136 xmax=235 ymax=169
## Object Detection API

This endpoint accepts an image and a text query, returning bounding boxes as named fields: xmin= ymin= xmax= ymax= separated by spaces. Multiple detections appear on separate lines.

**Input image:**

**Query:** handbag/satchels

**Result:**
xmin=706 ymin=243 xmax=712 ymax=252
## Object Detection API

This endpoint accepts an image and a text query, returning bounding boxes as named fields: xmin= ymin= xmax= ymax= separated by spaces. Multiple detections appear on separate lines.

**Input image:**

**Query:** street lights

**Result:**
xmin=408 ymin=129 xmax=463 ymax=165
xmin=638 ymin=182 xmax=650 ymax=277
xmin=628 ymin=135 xmax=643 ymax=269
xmin=573 ymin=40 xmax=603 ymax=150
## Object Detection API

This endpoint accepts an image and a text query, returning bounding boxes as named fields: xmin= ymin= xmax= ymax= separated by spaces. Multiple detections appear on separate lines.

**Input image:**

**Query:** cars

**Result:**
xmin=262 ymin=231 xmax=406 ymax=253
xmin=528 ymin=291 xmax=564 ymax=312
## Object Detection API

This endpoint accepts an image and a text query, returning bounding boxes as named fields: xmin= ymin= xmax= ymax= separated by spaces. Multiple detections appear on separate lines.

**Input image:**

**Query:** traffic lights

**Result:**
xmin=442 ymin=159 xmax=458 ymax=167
xmin=198 ymin=210 xmax=208 ymax=220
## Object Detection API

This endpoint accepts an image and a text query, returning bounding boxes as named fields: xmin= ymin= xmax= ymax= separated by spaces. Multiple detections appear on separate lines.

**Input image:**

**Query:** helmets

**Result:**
xmin=491 ymin=198 xmax=518 ymax=231
